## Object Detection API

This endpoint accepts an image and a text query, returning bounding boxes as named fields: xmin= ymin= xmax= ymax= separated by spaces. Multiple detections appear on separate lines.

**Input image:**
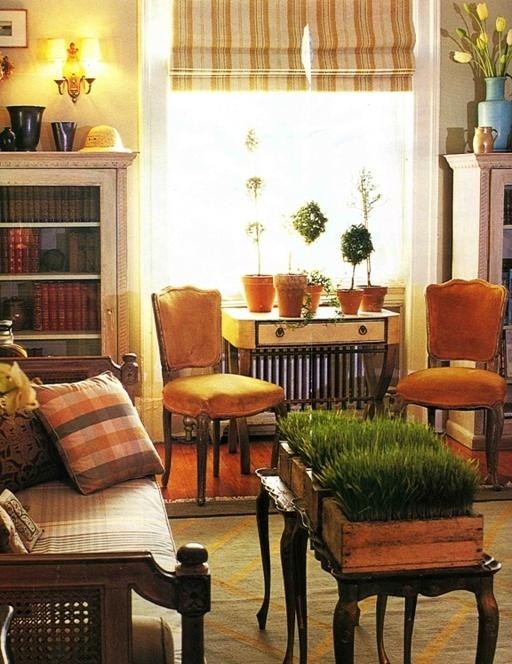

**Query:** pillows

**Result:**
xmin=30 ymin=369 xmax=166 ymax=496
xmin=0 ymin=410 xmax=64 ymax=494
xmin=6 ymin=615 xmax=175 ymax=664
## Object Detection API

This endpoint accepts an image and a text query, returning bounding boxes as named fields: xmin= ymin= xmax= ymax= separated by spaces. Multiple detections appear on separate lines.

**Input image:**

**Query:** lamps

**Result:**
xmin=44 ymin=38 xmax=102 ymax=103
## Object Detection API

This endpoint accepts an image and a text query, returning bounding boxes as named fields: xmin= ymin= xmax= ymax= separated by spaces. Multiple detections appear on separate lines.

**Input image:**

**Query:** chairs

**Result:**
xmin=152 ymin=287 xmax=288 ymax=506
xmin=0 ymin=604 xmax=15 ymax=664
xmin=392 ymin=279 xmax=508 ymax=489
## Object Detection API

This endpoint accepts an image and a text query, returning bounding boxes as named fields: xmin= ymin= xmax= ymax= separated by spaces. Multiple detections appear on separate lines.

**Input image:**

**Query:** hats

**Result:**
xmin=76 ymin=124 xmax=132 ymax=152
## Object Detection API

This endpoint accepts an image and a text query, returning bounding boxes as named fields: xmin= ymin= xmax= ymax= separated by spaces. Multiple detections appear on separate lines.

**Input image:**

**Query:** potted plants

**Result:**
xmin=241 ymin=128 xmax=276 ymax=312
xmin=351 ymin=168 xmax=388 ymax=312
xmin=277 ymin=411 xmax=484 ymax=572
xmin=275 ymin=210 xmax=307 ymax=318
xmin=336 ymin=224 xmax=374 ymax=314
xmin=290 ymin=200 xmax=328 ymax=315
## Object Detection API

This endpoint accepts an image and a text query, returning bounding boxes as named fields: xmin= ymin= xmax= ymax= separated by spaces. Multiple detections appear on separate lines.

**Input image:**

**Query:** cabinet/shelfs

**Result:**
xmin=444 ymin=153 xmax=512 ymax=449
xmin=0 ymin=151 xmax=140 ymax=366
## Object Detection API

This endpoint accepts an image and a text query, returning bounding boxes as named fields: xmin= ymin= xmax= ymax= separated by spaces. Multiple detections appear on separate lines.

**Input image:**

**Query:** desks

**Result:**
xmin=220 ymin=307 xmax=401 ymax=475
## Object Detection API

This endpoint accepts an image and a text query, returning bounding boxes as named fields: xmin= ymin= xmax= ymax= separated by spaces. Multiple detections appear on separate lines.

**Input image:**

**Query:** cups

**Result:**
xmin=472 ymin=124 xmax=498 ymax=154
xmin=49 ymin=121 xmax=77 ymax=152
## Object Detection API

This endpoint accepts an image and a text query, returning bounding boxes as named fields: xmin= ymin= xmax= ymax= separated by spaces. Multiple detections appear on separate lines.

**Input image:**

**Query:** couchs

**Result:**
xmin=0 ymin=354 xmax=210 ymax=664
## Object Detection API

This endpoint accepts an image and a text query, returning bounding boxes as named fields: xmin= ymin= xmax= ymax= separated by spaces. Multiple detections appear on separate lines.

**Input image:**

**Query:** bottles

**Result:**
xmin=0 ymin=126 xmax=17 ymax=152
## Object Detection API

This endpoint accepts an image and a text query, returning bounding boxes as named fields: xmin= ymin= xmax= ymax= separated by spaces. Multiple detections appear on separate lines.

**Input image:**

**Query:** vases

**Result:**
xmin=477 ymin=78 xmax=511 ymax=150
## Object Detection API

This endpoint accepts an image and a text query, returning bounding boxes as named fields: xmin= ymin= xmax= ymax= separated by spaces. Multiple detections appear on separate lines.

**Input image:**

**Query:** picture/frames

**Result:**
xmin=0 ymin=8 xmax=29 ymax=48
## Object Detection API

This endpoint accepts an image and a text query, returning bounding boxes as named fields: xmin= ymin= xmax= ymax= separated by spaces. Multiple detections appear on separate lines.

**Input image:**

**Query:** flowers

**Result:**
xmin=452 ymin=2 xmax=512 ymax=76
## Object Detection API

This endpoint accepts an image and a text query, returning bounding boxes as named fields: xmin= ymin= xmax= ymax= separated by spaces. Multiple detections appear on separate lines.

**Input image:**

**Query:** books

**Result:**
xmin=0 ymin=228 xmax=100 ymax=274
xmin=2 ymin=187 xmax=100 ymax=221
xmin=29 ymin=281 xmax=99 ymax=332
